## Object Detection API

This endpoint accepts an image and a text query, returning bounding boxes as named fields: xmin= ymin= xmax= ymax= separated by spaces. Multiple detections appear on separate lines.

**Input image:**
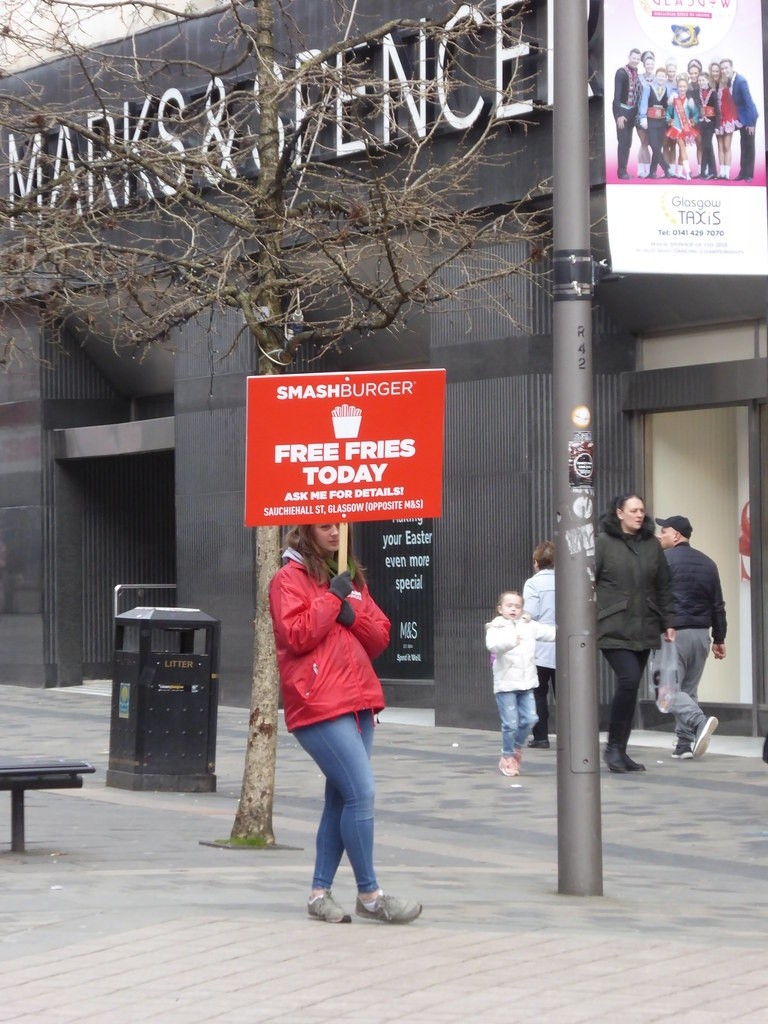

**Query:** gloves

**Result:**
xmin=336 ymin=600 xmax=355 ymax=626
xmin=329 ymin=571 xmax=352 ymax=600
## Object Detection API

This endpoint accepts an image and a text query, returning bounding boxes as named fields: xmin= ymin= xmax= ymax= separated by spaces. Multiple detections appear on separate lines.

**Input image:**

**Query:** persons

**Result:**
xmin=595 ymin=492 xmax=678 ymax=773
xmin=269 ymin=521 xmax=422 ymax=924
xmin=613 ymin=49 xmax=758 ymax=182
xmin=486 ymin=591 xmax=556 ymax=777
xmin=656 ymin=516 xmax=728 ymax=759
xmin=523 ymin=541 xmax=555 ymax=749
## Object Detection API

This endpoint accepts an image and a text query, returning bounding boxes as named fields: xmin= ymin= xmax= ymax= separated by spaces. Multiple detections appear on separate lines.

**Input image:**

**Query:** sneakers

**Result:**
xmin=308 ymin=891 xmax=351 ymax=923
xmin=498 ymin=756 xmax=519 ymax=776
xmin=356 ymin=889 xmax=422 ymax=924
xmin=514 ymin=747 xmax=521 ymax=760
xmin=693 ymin=716 xmax=719 ymax=756
xmin=672 ymin=737 xmax=694 ymax=759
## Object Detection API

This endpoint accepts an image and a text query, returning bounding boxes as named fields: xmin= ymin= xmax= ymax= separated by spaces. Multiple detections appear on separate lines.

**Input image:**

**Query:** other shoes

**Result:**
xmin=637 ymin=164 xmax=752 ymax=182
xmin=618 ymin=174 xmax=631 ymax=179
xmin=528 ymin=738 xmax=549 ymax=748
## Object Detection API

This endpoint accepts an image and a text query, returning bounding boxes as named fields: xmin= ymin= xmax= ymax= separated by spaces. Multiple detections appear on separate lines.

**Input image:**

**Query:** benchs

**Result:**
xmin=0 ymin=756 xmax=97 ymax=853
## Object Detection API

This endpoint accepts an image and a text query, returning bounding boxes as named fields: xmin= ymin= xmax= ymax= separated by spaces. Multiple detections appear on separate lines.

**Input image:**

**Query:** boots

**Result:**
xmin=619 ymin=722 xmax=646 ymax=771
xmin=603 ymin=722 xmax=626 ymax=772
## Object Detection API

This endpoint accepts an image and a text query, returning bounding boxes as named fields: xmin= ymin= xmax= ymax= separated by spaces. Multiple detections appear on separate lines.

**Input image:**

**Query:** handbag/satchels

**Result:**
xmin=656 ymin=638 xmax=680 ymax=713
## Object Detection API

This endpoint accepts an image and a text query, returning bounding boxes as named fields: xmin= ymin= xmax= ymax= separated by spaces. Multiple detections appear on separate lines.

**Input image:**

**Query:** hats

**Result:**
xmin=655 ymin=515 xmax=692 ymax=538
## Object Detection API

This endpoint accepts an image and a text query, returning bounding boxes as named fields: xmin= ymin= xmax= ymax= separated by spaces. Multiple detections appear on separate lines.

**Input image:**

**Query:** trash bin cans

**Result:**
xmin=104 ymin=607 xmax=222 ymax=794
